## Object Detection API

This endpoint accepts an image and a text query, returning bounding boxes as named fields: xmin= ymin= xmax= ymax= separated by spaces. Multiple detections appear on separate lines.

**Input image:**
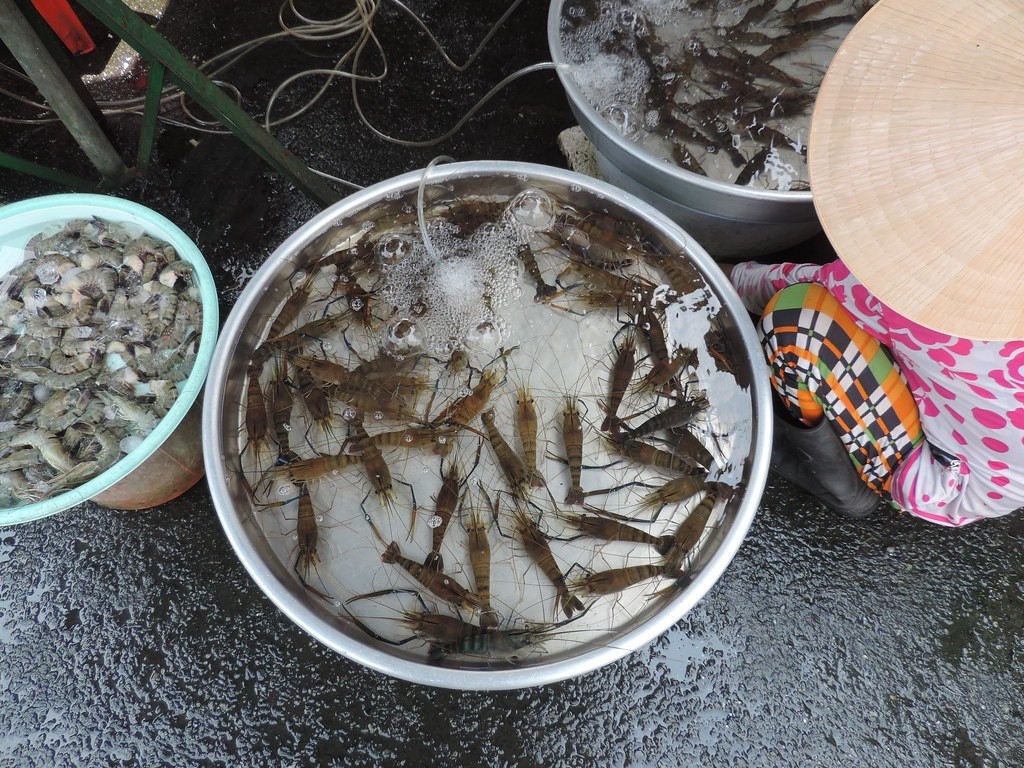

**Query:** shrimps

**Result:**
xmin=0 ymin=212 xmax=205 ymax=511
xmin=222 ymin=169 xmax=756 ymax=670
xmin=557 ymin=0 xmax=879 ymax=194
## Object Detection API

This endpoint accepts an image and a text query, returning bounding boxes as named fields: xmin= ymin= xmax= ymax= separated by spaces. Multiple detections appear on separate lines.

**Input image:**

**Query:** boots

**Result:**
xmin=771 ymin=410 xmax=882 ymax=518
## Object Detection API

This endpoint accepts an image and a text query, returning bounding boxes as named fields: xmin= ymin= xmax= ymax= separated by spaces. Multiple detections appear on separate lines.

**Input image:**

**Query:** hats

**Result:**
xmin=805 ymin=0 xmax=1024 ymax=342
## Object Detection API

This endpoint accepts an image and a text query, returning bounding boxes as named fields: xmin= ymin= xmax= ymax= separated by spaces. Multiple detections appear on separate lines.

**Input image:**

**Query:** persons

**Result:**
xmin=713 ymin=0 xmax=1024 ymax=526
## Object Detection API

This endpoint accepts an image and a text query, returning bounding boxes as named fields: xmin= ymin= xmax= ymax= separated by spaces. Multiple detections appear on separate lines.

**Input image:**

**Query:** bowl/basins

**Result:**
xmin=0 ymin=193 xmax=219 ymax=526
xmin=204 ymin=161 xmax=771 ymax=691
xmin=549 ymin=0 xmax=886 ymax=226
